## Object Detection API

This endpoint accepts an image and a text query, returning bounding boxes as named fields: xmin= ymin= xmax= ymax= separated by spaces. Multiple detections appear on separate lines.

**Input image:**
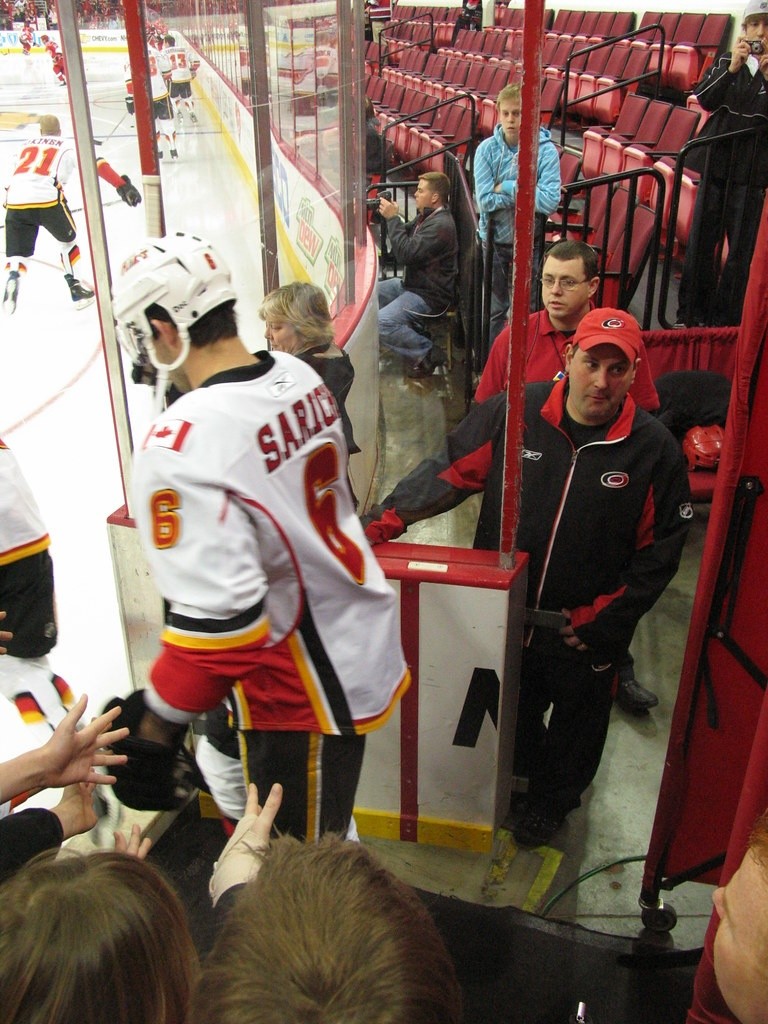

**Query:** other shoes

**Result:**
xmin=409 ymin=346 xmax=446 ymax=377
xmin=513 ymin=811 xmax=560 ymax=847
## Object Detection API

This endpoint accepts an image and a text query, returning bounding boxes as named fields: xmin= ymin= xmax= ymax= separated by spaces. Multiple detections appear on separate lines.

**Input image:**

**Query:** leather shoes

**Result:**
xmin=616 ymin=680 xmax=658 ymax=709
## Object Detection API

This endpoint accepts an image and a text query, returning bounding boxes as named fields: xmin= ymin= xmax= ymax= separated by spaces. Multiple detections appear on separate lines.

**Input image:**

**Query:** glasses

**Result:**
xmin=540 ymin=277 xmax=589 ymax=290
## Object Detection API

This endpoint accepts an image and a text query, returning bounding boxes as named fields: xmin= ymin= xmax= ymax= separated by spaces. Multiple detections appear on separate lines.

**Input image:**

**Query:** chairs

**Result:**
xmin=362 ymin=6 xmax=734 ymax=327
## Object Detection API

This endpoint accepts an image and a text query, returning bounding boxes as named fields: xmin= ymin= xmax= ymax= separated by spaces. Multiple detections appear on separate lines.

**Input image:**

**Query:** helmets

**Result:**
xmin=109 ymin=231 xmax=237 ymax=366
xmin=41 ymin=35 xmax=49 ymax=42
xmin=682 ymin=424 xmax=725 ymax=472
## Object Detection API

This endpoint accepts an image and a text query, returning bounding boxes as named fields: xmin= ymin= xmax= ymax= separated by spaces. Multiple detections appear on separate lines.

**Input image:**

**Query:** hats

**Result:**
xmin=572 ymin=307 xmax=642 ymax=363
xmin=743 ymin=0 xmax=768 ymax=18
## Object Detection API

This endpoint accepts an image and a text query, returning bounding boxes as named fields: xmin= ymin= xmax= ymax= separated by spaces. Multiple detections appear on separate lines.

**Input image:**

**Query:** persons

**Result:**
xmin=451 ymin=0 xmax=482 ymax=45
xmin=77 ymin=0 xmax=174 ymax=50
xmin=355 ymin=307 xmax=692 ymax=848
xmin=368 ymin=0 xmax=395 ymax=43
xmin=1 ymin=695 xmax=467 ymax=1023
xmin=0 ymin=0 xmax=58 ymax=28
xmin=258 ymin=284 xmax=362 ymax=456
xmin=40 ymin=35 xmax=66 ymax=86
xmin=19 ymin=28 xmax=33 ymax=55
xmin=473 ymin=83 xmax=562 ymax=352
xmin=476 ymin=242 xmax=661 ymax=714
xmin=689 ymin=814 xmax=768 ymax=1024
xmin=0 ymin=438 xmax=92 ymax=845
xmin=124 ymin=44 xmax=178 ymax=160
xmin=91 ymin=229 xmax=414 ymax=840
xmin=366 ymin=96 xmax=389 ymax=173
xmin=0 ymin=112 xmax=141 ymax=315
xmin=674 ymin=1 xmax=768 ymax=334
xmin=159 ymin=36 xmax=201 ymax=126
xmin=379 ymin=172 xmax=459 ymax=377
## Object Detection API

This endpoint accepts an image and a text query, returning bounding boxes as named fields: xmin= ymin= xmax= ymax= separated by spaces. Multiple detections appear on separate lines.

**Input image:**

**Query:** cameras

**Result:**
xmin=743 ymin=39 xmax=764 ymax=55
xmin=366 ymin=191 xmax=392 ymax=211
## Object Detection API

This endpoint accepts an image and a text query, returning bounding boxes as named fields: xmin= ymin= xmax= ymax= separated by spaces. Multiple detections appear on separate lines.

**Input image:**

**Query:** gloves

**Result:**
xmin=116 ymin=175 xmax=142 ymax=207
xmin=126 ymin=97 xmax=134 ymax=115
xmin=102 ymin=687 xmax=190 ymax=811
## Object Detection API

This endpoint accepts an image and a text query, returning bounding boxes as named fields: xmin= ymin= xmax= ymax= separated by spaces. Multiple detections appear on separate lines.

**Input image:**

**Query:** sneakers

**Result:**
xmin=170 ymin=149 xmax=178 ymax=161
xmin=3 ymin=271 xmax=20 ymax=313
xmin=190 ymin=113 xmax=197 ymax=123
xmin=159 ymin=150 xmax=164 ymax=162
xmin=177 ymin=113 xmax=184 ymax=124
xmin=65 ymin=274 xmax=95 ymax=310
